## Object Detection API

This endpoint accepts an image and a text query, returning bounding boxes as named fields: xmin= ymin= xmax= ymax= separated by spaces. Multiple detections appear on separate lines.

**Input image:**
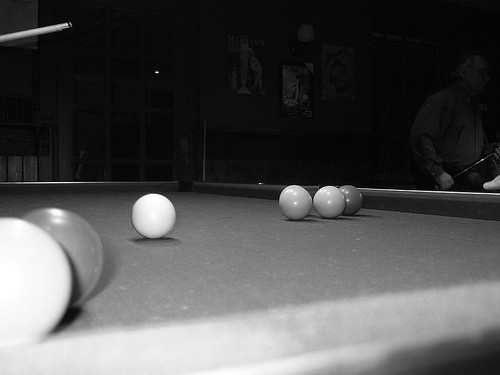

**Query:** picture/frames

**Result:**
xmin=323 ymin=42 xmax=357 ymax=104
xmin=224 ymin=31 xmax=275 ymax=101
xmin=277 ymin=60 xmax=316 ymax=120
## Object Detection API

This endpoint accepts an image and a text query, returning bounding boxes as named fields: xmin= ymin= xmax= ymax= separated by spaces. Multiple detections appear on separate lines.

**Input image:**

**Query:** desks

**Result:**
xmin=1 ymin=176 xmax=499 ymax=375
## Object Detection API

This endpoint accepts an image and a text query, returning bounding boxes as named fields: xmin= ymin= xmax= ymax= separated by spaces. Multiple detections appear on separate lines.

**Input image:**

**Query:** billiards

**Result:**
xmin=278 ymin=185 xmax=313 ymax=221
xmin=313 ymin=185 xmax=346 ymax=218
xmin=130 ymin=193 xmax=177 ymax=239
xmin=18 ymin=208 xmax=103 ymax=308
xmin=338 ymin=185 xmax=363 ymax=216
xmin=0 ymin=216 xmax=73 ymax=346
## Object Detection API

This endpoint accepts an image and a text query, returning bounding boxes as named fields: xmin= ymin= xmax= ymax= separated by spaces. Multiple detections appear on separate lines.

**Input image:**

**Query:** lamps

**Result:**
xmin=288 ymin=23 xmax=315 ymax=53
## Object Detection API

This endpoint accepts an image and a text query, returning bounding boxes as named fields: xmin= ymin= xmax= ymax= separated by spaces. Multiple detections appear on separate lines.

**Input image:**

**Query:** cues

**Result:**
xmin=1 ymin=22 xmax=73 ymax=42
xmin=202 ymin=119 xmax=207 ymax=182
xmin=433 ymin=144 xmax=500 ymax=190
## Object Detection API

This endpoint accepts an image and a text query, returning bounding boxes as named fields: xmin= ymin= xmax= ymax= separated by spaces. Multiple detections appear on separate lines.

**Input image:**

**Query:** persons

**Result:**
xmin=409 ymin=52 xmax=500 ymax=193
